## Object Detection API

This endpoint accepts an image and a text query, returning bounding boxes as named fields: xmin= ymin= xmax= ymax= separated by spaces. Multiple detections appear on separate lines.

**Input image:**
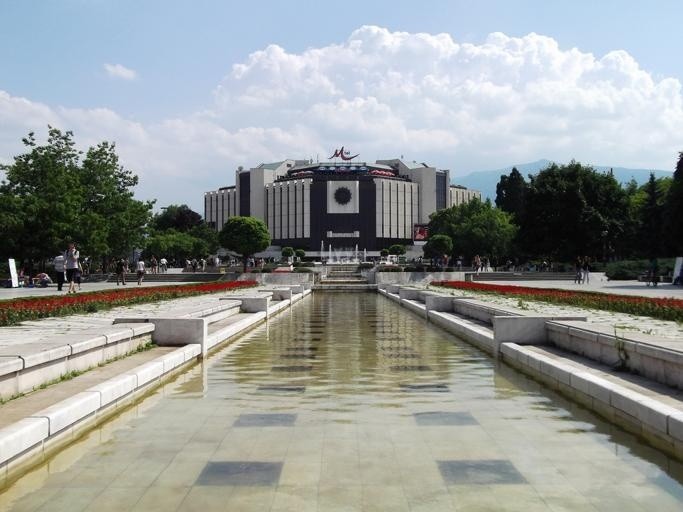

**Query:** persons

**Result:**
xmin=109 ymin=256 xmax=129 ymax=275
xmin=403 ymin=253 xmax=554 ymax=278
xmin=149 ymin=254 xmax=157 ymax=275
xmin=18 ymin=267 xmax=53 ymax=289
xmin=159 ymin=256 xmax=167 ymax=273
xmin=50 ymin=251 xmax=65 ymax=291
xmin=573 ymin=255 xmax=582 ymax=284
xmin=61 ymin=242 xmax=79 ymax=293
xmin=114 ymin=258 xmax=127 ymax=286
xmin=168 ymin=253 xmax=276 ymax=273
xmin=581 ymin=256 xmax=591 ymax=284
xmin=72 ymin=260 xmax=82 ymax=291
xmin=646 ymin=256 xmax=657 ymax=288
xmin=134 ymin=256 xmax=145 ymax=286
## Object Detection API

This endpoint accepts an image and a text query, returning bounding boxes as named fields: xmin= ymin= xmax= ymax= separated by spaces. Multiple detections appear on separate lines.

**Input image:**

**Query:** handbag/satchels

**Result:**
xmin=139 ymin=270 xmax=146 ymax=275
